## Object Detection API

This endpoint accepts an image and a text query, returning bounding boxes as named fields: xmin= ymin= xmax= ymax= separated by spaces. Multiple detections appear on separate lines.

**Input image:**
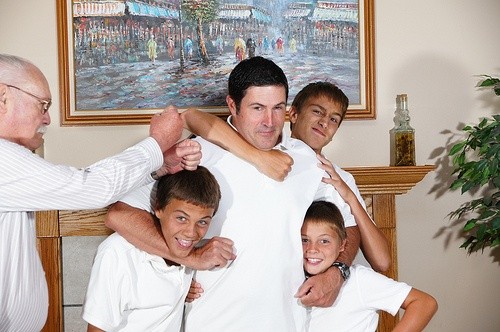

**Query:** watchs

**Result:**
xmin=332 ymin=262 xmax=350 ymax=281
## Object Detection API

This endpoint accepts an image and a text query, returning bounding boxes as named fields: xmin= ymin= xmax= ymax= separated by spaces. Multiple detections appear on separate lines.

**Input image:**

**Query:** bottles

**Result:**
xmin=394 ymin=94 xmax=416 ymax=166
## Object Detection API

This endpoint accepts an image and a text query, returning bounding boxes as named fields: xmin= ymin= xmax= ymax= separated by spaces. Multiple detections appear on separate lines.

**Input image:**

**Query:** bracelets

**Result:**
xmin=149 ymin=170 xmax=161 ymax=180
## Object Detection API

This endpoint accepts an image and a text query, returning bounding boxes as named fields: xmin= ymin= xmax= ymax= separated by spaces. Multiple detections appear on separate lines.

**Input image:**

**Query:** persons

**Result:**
xmin=180 ymin=81 xmax=391 ymax=273
xmin=184 ymin=201 xmax=438 ymax=332
xmin=104 ymin=56 xmax=361 ymax=332
xmin=0 ymin=53 xmax=202 ymax=332
xmin=83 ymin=164 xmax=221 ymax=332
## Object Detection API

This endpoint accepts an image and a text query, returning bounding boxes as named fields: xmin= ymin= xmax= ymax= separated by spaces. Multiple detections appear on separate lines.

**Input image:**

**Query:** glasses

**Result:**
xmin=6 ymin=84 xmax=53 ymax=115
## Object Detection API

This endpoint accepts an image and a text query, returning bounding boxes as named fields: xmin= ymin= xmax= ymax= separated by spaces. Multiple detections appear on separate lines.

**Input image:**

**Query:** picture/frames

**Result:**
xmin=56 ymin=0 xmax=376 ymax=127
